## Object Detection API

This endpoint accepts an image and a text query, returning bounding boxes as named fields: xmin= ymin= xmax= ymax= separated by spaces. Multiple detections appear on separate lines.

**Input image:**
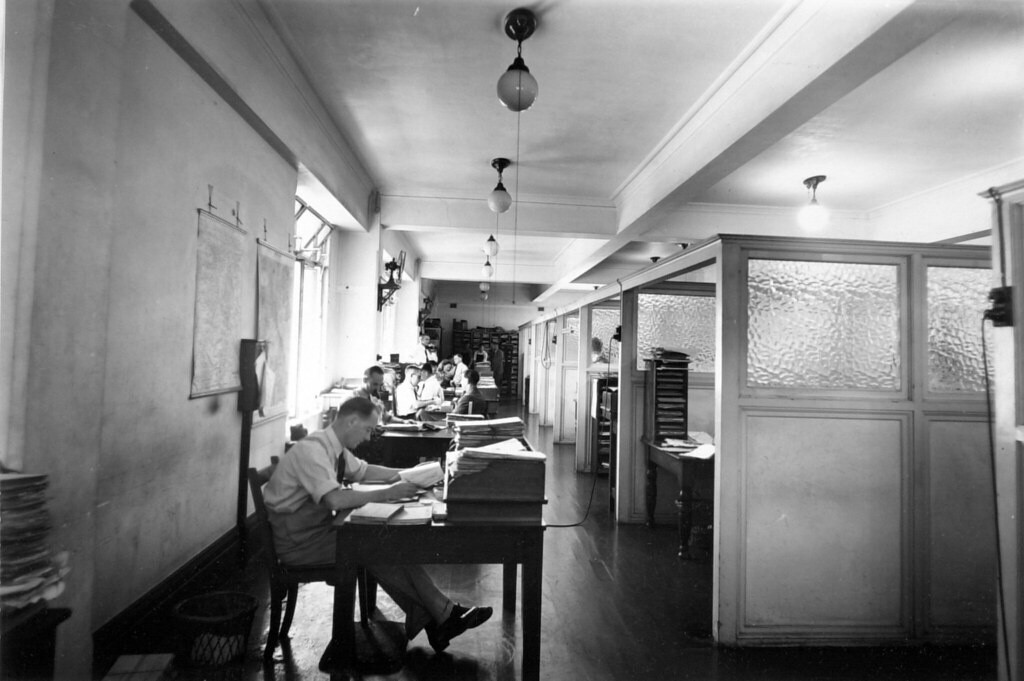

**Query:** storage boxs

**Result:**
xmin=101 ymin=653 xmax=186 ymax=681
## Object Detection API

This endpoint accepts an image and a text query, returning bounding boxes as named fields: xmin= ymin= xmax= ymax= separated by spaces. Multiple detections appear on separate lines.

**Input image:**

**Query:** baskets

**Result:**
xmin=175 ymin=590 xmax=259 ymax=681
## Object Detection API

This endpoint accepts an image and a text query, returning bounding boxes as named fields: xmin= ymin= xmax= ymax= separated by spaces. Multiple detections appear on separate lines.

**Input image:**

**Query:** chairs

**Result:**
xmin=247 ymin=456 xmax=372 ymax=663
xmin=468 ymin=400 xmax=489 ymax=422
xmin=391 ymin=387 xmax=424 ymax=421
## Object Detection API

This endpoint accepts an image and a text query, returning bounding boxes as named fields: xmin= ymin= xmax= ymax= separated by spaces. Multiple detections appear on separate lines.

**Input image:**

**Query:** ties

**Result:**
xmin=425 ymin=350 xmax=428 ymax=362
xmin=414 ymin=388 xmax=417 ymax=401
xmin=337 ymin=451 xmax=346 ymax=483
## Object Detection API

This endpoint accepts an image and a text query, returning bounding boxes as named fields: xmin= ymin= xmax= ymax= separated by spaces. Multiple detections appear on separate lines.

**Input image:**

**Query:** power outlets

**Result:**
xmin=615 ymin=326 xmax=621 ymax=342
xmin=987 ymin=285 xmax=1014 ymax=328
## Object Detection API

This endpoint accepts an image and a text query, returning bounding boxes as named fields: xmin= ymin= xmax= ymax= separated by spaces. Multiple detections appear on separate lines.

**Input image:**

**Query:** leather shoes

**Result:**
xmin=427 ymin=602 xmax=492 ymax=652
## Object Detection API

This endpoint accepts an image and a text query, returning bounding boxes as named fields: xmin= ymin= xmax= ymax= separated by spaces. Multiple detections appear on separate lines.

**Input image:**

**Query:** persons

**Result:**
xmin=356 ymin=342 xmax=505 ymax=467
xmin=262 ymin=394 xmax=494 ymax=653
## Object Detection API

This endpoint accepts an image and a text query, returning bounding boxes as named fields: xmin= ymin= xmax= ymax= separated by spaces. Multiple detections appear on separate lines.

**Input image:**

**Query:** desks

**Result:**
xmin=416 ymin=400 xmax=455 ymax=422
xmin=442 ymin=376 xmax=498 ymax=421
xmin=641 ymin=436 xmax=715 ymax=563
xmin=0 ymin=602 xmax=73 ymax=681
xmin=330 ymin=468 xmax=544 ymax=681
xmin=377 ymin=418 xmax=490 ymax=470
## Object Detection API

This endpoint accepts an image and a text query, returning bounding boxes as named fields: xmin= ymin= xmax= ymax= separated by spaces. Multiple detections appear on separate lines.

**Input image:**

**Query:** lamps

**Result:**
xmin=480 ymin=282 xmax=490 ymax=292
xmin=484 ymin=235 xmax=499 ymax=256
xmin=377 ymin=250 xmax=407 ymax=313
xmin=482 ymin=255 xmax=494 ymax=278
xmin=418 ymin=295 xmax=434 ymax=327
xmin=479 ymin=291 xmax=488 ymax=301
xmin=800 ymin=176 xmax=828 ymax=233
xmin=487 ymin=156 xmax=512 ymax=213
xmin=494 ymin=9 xmax=542 ymax=115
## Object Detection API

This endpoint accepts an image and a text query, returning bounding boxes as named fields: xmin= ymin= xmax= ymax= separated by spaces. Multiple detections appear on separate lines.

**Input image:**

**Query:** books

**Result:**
xmin=352 ymin=376 xmax=547 ymax=524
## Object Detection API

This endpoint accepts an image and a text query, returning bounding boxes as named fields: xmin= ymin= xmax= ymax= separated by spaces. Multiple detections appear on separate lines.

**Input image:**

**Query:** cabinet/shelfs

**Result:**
xmin=453 ymin=329 xmax=519 ymax=398
xmin=590 ymin=376 xmax=619 ymax=475
xmin=643 ymin=358 xmax=692 ymax=444
